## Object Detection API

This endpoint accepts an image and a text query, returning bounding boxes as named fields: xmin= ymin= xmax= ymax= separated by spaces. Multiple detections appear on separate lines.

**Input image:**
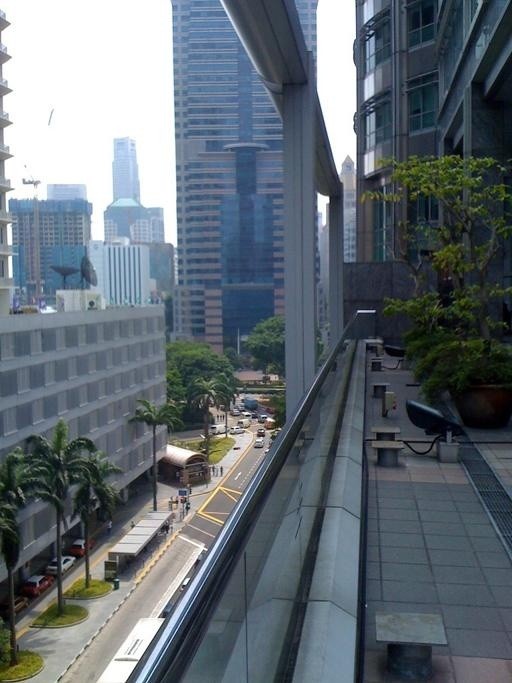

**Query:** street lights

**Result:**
xmin=186 ymin=503 xmax=190 ymax=509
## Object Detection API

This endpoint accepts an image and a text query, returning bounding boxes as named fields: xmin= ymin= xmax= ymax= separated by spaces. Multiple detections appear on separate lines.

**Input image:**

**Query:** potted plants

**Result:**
xmin=360 ymin=155 xmax=511 ymax=430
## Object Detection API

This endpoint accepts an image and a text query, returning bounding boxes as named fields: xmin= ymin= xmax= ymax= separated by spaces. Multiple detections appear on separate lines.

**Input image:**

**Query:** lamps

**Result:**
xmin=405 ymin=398 xmax=468 ymax=463
xmin=384 ymin=344 xmax=410 ymax=369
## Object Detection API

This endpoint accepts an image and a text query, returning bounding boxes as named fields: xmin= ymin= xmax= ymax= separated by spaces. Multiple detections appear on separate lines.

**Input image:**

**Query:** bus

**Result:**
xmin=243 ymin=398 xmax=259 ymax=409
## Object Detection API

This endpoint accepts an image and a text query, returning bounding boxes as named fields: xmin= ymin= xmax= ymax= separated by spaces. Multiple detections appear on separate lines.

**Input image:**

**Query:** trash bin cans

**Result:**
xmin=113 ymin=579 xmax=120 ymax=589
xmin=186 ymin=503 xmax=190 ymax=509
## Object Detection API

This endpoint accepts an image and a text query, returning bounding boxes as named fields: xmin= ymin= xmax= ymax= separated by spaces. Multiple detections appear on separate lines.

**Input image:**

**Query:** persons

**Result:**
xmin=211 ymin=464 xmax=223 ymax=477
xmin=217 ymin=413 xmax=224 ymax=421
xmin=106 ymin=518 xmax=112 ymax=534
xmin=131 ymin=521 xmax=135 ymax=528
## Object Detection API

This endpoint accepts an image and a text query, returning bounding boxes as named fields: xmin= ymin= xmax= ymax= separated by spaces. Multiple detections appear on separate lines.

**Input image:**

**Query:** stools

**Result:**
xmin=370 ymin=381 xmax=391 ymax=398
xmin=374 ymin=611 xmax=449 ymax=681
xmin=371 ymin=425 xmax=405 ymax=465
xmin=370 ymin=356 xmax=384 ymax=370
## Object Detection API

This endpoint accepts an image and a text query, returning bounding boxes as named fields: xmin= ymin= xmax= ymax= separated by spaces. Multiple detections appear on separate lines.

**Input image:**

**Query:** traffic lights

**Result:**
xmin=189 ymin=488 xmax=192 ymax=494
xmin=181 ymin=497 xmax=187 ymax=503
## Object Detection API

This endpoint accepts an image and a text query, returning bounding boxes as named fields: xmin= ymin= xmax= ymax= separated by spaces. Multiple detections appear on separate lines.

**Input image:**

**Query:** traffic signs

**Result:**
xmin=179 ymin=490 xmax=186 ymax=496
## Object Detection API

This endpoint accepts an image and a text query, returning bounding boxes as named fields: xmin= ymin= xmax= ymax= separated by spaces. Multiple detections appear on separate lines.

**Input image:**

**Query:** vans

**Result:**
xmin=210 ymin=403 xmax=282 ymax=448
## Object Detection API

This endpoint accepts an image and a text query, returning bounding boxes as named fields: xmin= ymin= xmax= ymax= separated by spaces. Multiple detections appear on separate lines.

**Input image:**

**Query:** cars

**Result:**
xmin=1 ymin=536 xmax=95 ymax=620
